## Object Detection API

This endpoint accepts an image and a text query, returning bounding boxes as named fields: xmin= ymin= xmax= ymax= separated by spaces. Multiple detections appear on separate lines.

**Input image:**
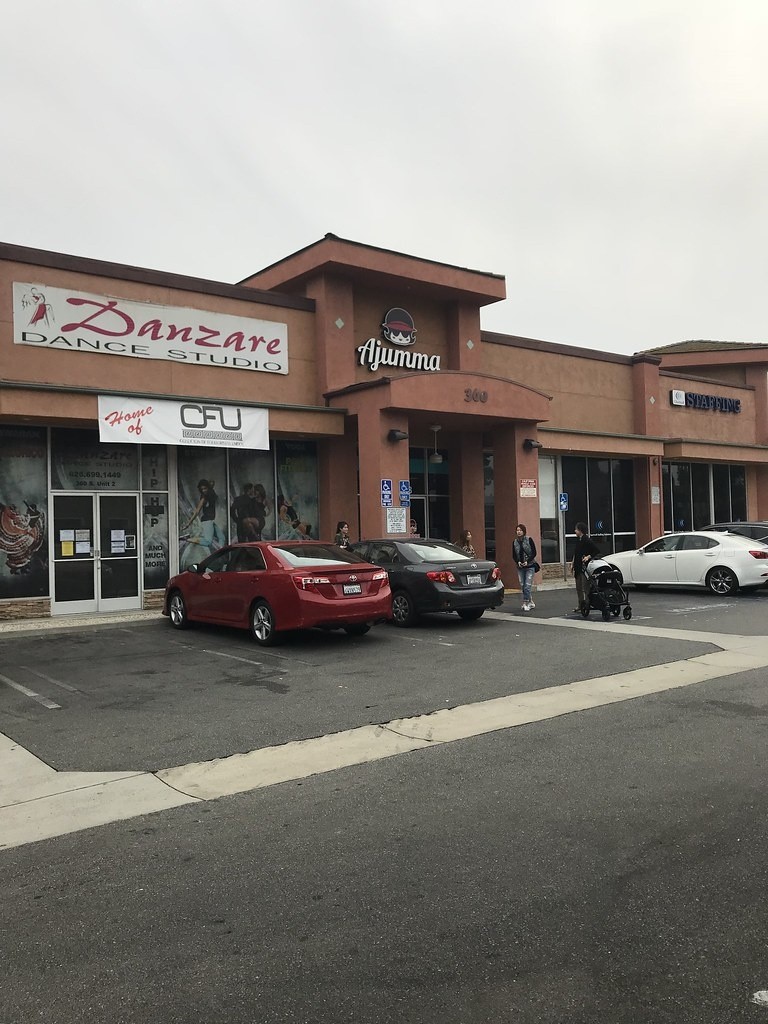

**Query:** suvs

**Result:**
xmin=698 ymin=522 xmax=768 ymax=550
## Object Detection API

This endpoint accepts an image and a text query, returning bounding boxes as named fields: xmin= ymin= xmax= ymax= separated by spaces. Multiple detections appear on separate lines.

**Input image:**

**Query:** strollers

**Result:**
xmin=579 ymin=556 xmax=632 ymax=622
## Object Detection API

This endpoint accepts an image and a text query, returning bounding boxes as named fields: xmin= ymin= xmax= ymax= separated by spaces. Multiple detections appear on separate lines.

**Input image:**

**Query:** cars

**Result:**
xmin=340 ymin=538 xmax=506 ymax=629
xmin=600 ymin=532 xmax=768 ymax=596
xmin=161 ymin=539 xmax=394 ymax=648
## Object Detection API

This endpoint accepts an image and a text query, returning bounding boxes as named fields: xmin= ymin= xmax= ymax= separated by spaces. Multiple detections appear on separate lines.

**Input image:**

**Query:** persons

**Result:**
xmin=180 ymin=479 xmax=218 ymax=571
xmin=512 ymin=524 xmax=540 ymax=611
xmin=410 ymin=519 xmax=416 ymax=535
xmin=454 ymin=529 xmax=476 ymax=559
xmin=333 ymin=521 xmax=350 ymax=550
xmin=1 ymin=500 xmax=45 ymax=576
xmin=277 ymin=494 xmax=312 ymax=540
xmin=568 ymin=522 xmax=595 ymax=613
xmin=230 ymin=483 xmax=273 ymax=543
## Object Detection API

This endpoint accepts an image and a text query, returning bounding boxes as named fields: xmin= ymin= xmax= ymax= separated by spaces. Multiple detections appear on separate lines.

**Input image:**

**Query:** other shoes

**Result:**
xmin=572 ymin=604 xmax=583 ymax=612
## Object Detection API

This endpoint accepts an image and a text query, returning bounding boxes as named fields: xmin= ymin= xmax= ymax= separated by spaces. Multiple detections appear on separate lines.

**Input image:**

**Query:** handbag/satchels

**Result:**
xmin=533 ymin=558 xmax=541 ymax=573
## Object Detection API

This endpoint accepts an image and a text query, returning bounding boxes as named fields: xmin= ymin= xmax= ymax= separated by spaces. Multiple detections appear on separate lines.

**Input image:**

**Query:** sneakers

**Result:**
xmin=529 ymin=602 xmax=535 ymax=609
xmin=522 ymin=603 xmax=530 ymax=611
xmin=178 ymin=533 xmax=191 ymax=541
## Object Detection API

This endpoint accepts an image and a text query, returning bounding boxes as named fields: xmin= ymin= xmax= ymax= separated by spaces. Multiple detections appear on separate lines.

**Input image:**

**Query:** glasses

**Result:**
xmin=409 ymin=524 xmax=415 ymax=528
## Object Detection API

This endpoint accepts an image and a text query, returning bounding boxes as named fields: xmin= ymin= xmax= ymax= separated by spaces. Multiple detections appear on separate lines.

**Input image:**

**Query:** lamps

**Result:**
xmin=430 ymin=424 xmax=442 ymax=464
